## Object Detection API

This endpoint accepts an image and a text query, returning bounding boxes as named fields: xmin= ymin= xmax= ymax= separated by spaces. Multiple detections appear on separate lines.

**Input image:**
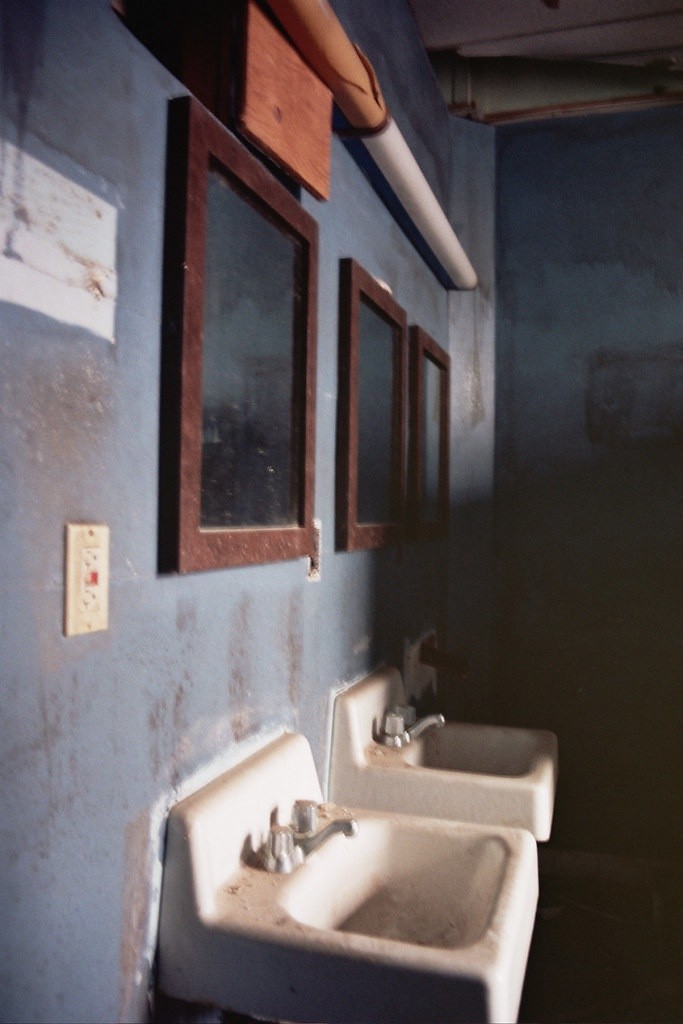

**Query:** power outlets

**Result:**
xmin=64 ymin=523 xmax=109 ymax=637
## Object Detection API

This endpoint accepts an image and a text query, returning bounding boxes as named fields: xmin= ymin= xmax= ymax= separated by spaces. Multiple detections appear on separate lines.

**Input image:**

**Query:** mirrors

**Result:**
xmin=172 ymin=95 xmax=528 ymax=573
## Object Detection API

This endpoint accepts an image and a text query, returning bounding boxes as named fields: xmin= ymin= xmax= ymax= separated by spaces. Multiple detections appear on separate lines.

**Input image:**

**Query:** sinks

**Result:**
xmin=260 ymin=810 xmax=540 ymax=1024
xmin=400 ymin=719 xmax=560 ymax=841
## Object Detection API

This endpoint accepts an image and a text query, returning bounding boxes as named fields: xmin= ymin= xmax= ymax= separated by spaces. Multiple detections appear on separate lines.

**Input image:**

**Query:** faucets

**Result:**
xmin=384 ymin=710 xmax=447 ymax=747
xmin=264 ymin=799 xmax=359 ymax=876
